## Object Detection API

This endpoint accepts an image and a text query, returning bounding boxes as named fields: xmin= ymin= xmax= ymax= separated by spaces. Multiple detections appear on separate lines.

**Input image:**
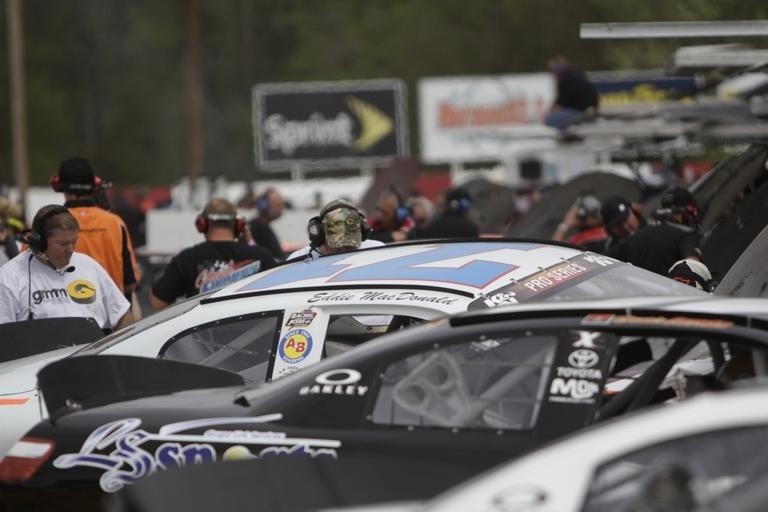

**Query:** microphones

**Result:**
xmin=102 ymin=182 xmax=113 ymax=190
xmin=311 ymin=246 xmax=324 ymax=259
xmin=36 ymin=249 xmax=75 ymax=277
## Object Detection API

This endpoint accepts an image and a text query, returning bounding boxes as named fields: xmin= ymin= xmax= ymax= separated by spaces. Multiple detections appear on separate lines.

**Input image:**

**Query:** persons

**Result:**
xmin=547 ymin=58 xmax=605 ymax=128
xmin=1 ymin=155 xmax=715 ymax=333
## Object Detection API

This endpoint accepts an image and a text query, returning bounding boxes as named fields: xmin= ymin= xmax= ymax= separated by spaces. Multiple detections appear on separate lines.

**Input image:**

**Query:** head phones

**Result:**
xmin=308 ymin=202 xmax=373 ymax=244
xmin=257 ymin=188 xmax=272 ymax=211
xmin=194 ymin=212 xmax=247 ymax=236
xmin=49 ymin=173 xmax=102 ymax=197
xmin=577 ymin=194 xmax=588 ymax=219
xmin=28 ymin=205 xmax=69 ymax=254
xmin=603 ymin=201 xmax=642 ymax=238
xmin=396 ymin=209 xmax=409 ymax=224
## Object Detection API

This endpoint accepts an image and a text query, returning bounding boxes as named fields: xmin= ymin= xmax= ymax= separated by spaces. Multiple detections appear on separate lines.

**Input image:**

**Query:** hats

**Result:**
xmin=660 ymin=187 xmax=692 ymax=210
xmin=667 ymin=259 xmax=713 ymax=291
xmin=320 ymin=199 xmax=362 ymax=249
xmin=601 ymin=196 xmax=630 ymax=225
